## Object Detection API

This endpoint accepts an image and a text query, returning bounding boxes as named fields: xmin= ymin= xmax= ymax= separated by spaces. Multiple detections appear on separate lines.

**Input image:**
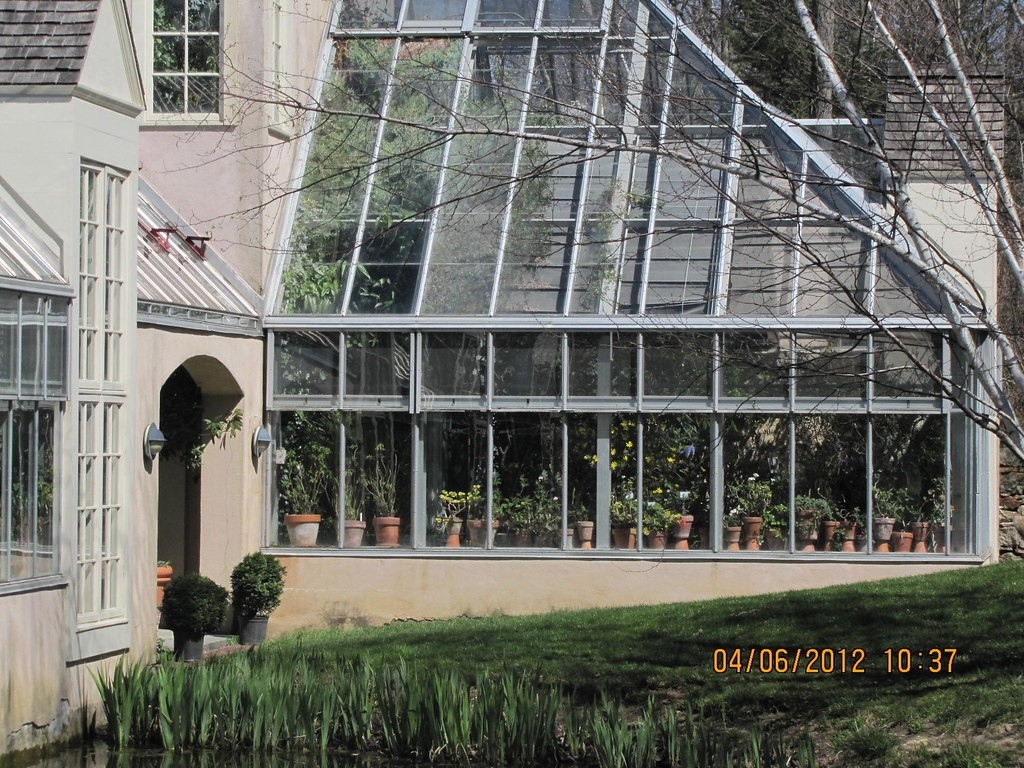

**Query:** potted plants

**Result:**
xmin=433 ymin=468 xmax=946 ymax=553
xmin=280 ymin=438 xmax=330 ymax=548
xmin=333 ymin=442 xmax=370 ymax=549
xmin=155 ymin=560 xmax=174 ymax=608
xmin=358 ymin=442 xmax=403 ymax=547
xmin=230 ymin=551 xmax=288 ymax=647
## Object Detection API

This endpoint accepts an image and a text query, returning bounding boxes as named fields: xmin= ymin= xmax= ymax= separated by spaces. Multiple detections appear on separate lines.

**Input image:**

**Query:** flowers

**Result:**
xmin=724 ymin=503 xmax=747 ymax=524
xmin=735 ymin=472 xmax=773 ymax=517
xmin=429 ymin=481 xmax=483 ymax=524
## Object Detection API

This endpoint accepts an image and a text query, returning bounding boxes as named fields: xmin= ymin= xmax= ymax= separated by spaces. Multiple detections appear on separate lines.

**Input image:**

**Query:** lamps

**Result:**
xmin=252 ymin=425 xmax=272 ymax=458
xmin=143 ymin=422 xmax=167 ymax=462
